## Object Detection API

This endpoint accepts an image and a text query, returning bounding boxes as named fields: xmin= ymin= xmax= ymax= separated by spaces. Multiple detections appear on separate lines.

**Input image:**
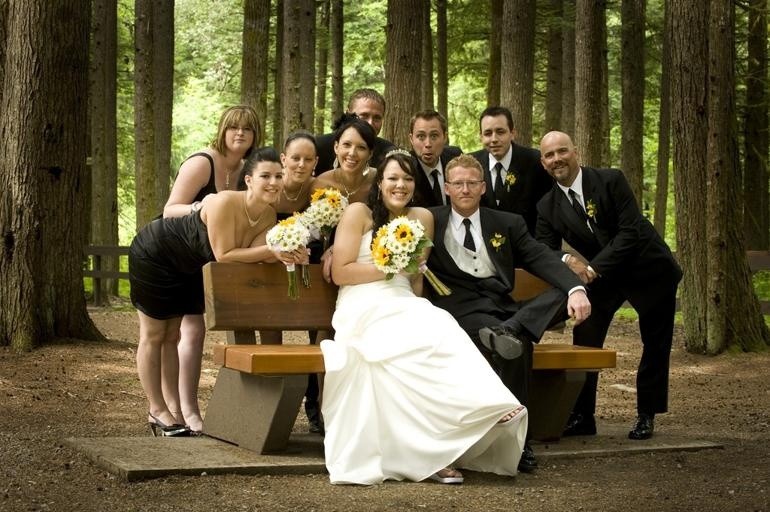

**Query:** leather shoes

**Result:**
xmin=519 ymin=443 xmax=538 ymax=470
xmin=628 ymin=413 xmax=654 ymax=439
xmin=478 ymin=326 xmax=523 ymax=361
xmin=562 ymin=413 xmax=597 ymax=436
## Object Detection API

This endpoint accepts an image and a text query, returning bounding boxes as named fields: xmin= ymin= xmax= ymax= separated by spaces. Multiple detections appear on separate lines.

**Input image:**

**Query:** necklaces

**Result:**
xmin=282 ymin=183 xmax=304 ymax=202
xmin=243 ymin=198 xmax=265 ymax=228
xmin=341 ymin=173 xmax=367 ymax=201
xmin=224 ymin=170 xmax=231 ymax=189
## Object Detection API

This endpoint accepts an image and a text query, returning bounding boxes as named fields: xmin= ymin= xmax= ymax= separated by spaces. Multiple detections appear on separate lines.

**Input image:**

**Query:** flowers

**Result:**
xmin=369 ymin=213 xmax=455 ymax=297
xmin=294 ymin=209 xmax=316 ymax=290
xmin=307 ymin=184 xmax=351 ymax=251
xmin=265 ymin=210 xmax=304 ymax=300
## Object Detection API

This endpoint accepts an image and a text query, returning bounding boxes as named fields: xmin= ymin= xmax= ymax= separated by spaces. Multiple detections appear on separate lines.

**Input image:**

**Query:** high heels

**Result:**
xmin=148 ymin=412 xmax=191 ymax=437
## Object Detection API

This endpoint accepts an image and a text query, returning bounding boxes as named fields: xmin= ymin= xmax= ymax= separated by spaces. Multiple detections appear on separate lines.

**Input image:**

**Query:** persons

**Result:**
xmin=304 ymin=88 xmax=398 ymax=434
xmin=319 ymin=147 xmax=528 ymax=486
xmin=154 ymin=104 xmax=262 ymax=432
xmin=533 ymin=130 xmax=683 ymax=440
xmin=466 ymin=107 xmax=554 ymax=238
xmin=322 ymin=153 xmax=592 ymax=473
xmin=406 ymin=109 xmax=485 ymax=208
xmin=308 ymin=112 xmax=377 ymax=437
xmin=128 ymin=146 xmax=308 ymax=437
xmin=259 ymin=129 xmax=320 ymax=345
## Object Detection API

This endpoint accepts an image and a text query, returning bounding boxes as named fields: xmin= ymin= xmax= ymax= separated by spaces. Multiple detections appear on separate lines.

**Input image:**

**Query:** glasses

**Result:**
xmin=446 ymin=179 xmax=483 ymax=188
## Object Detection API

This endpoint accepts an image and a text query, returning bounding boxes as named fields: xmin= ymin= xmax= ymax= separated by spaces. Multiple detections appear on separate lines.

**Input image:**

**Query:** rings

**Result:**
xmin=585 ymin=312 xmax=589 ymax=315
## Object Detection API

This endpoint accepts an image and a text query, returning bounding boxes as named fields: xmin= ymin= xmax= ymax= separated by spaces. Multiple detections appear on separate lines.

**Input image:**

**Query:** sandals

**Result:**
xmin=429 ymin=465 xmax=464 ymax=484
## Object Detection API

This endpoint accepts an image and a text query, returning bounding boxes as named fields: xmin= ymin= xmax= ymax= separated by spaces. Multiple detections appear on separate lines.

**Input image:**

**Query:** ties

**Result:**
xmin=494 ymin=162 xmax=504 ymax=200
xmin=568 ymin=188 xmax=587 ymax=224
xmin=430 ymin=169 xmax=444 ymax=206
xmin=462 ymin=218 xmax=476 ymax=252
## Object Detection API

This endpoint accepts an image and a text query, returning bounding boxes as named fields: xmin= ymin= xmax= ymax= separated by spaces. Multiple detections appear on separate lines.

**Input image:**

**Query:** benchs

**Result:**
xmin=201 ymin=261 xmax=618 ymax=456
